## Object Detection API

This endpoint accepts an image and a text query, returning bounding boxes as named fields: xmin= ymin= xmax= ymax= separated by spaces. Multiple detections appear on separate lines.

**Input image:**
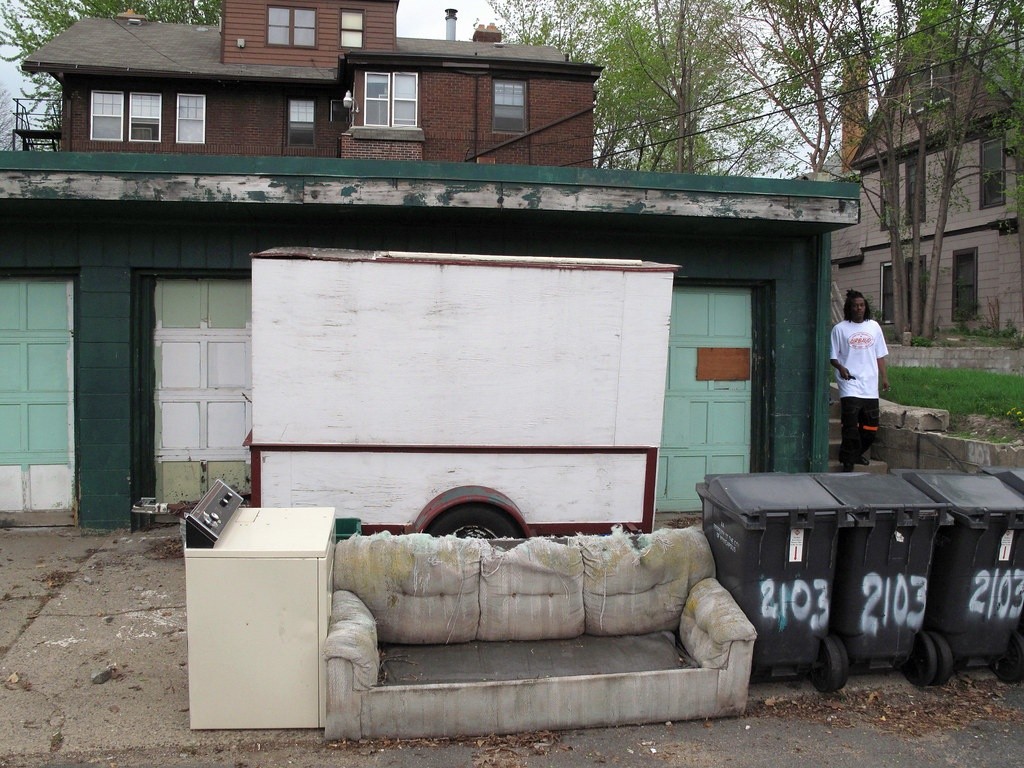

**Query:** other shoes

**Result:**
xmin=856 ymin=457 xmax=869 ymax=465
xmin=843 ymin=458 xmax=854 ymax=472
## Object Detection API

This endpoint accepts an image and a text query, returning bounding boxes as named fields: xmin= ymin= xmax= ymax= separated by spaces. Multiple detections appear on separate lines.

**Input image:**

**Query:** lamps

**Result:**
xmin=343 ymin=90 xmax=360 ymax=113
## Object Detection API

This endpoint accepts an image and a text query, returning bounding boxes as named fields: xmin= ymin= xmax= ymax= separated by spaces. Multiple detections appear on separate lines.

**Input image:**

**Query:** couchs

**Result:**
xmin=322 ymin=525 xmax=758 ymax=742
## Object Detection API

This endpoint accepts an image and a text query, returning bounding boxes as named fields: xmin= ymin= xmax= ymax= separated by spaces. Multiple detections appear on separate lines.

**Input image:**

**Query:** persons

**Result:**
xmin=830 ymin=288 xmax=891 ymax=472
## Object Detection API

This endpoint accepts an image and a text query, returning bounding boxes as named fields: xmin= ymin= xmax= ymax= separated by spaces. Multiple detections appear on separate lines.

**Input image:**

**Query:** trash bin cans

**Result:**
xmin=694 ymin=471 xmax=845 ymax=692
xmin=814 ymin=475 xmax=939 ymax=689
xmin=902 ymin=465 xmax=1024 ymax=685
